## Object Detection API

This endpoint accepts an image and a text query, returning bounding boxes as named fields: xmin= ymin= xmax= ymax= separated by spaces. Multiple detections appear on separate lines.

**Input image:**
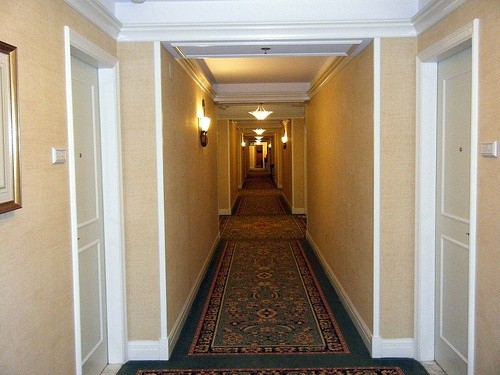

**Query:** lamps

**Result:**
xmin=247 ymin=103 xmax=274 ymax=120
xmin=282 ymin=136 xmax=288 ymax=150
xmin=200 ymin=116 xmax=211 ymax=147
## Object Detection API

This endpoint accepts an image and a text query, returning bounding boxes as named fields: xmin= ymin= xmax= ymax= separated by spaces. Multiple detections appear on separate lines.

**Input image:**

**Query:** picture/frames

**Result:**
xmin=0 ymin=41 xmax=23 ymax=214
